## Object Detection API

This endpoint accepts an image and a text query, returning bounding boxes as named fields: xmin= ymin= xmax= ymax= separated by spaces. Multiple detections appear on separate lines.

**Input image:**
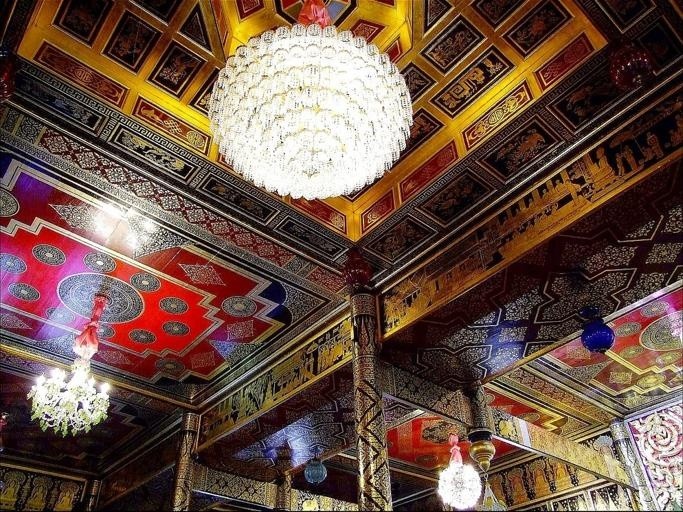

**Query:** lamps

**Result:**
xmin=304 ymin=448 xmax=327 ymax=484
xmin=208 ymin=0 xmax=414 ymax=201
xmin=27 ymin=295 xmax=110 ymax=438
xmin=437 ymin=433 xmax=482 ymax=509
xmin=579 ymin=307 xmax=615 ymax=355
